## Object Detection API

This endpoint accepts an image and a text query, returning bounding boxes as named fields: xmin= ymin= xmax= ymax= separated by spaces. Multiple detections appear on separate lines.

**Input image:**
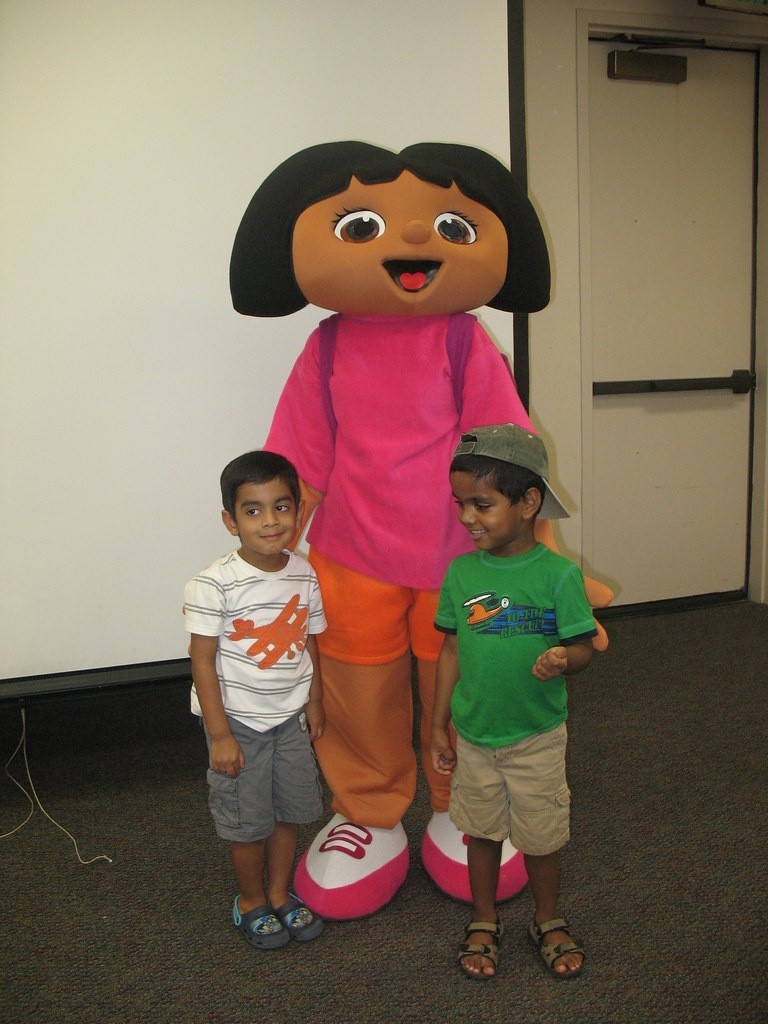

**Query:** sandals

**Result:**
xmin=268 ymin=891 xmax=323 ymax=942
xmin=458 ymin=912 xmax=504 ymax=981
xmin=232 ymin=895 xmax=290 ymax=949
xmin=527 ymin=912 xmax=585 ymax=978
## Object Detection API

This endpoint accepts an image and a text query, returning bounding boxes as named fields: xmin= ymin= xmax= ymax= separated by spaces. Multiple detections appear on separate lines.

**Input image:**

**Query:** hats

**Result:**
xmin=453 ymin=421 xmax=571 ymax=520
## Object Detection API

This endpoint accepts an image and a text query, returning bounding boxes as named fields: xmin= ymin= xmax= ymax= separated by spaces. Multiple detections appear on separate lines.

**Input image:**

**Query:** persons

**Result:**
xmin=181 ymin=449 xmax=329 ymax=947
xmin=430 ymin=423 xmax=598 ymax=981
xmin=229 ymin=140 xmax=617 ymax=920
